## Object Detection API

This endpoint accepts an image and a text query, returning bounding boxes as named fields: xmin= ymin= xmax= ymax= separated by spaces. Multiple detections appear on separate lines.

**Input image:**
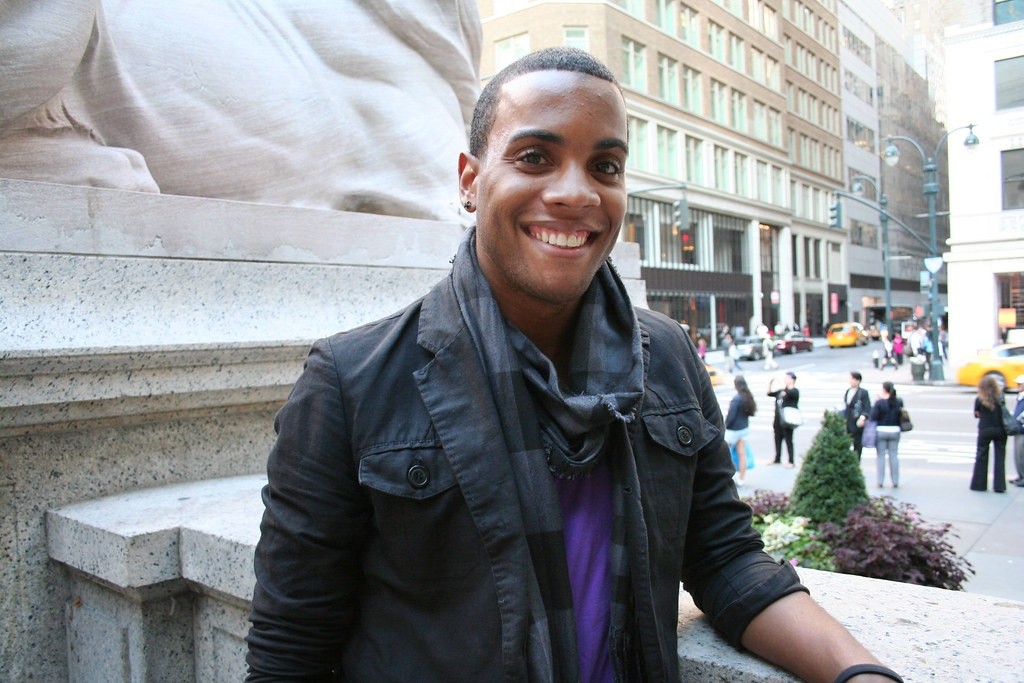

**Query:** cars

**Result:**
xmin=734 ymin=336 xmax=774 ymax=361
xmin=957 ymin=345 xmax=1024 ymax=395
xmin=774 ymin=332 xmax=813 ymax=354
xmin=827 ymin=322 xmax=869 ymax=348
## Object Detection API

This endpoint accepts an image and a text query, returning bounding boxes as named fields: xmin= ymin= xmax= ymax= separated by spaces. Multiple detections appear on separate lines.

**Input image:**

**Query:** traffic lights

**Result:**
xmin=830 ymin=203 xmax=842 ymax=230
xmin=673 ymin=197 xmax=689 ymax=232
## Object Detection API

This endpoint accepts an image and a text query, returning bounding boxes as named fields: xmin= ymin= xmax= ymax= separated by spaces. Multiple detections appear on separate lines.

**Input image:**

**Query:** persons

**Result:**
xmin=696 ymin=321 xmax=831 ymax=372
xmin=725 ymin=374 xmax=756 ymax=485
xmin=244 ymin=49 xmax=905 ymax=683
xmin=767 ymin=372 xmax=799 ymax=468
xmin=844 ymin=371 xmax=871 ymax=460
xmin=881 ymin=324 xmax=949 ymax=371
xmin=870 ymin=382 xmax=903 ymax=488
xmin=970 ymin=376 xmax=1008 ymax=492
xmin=1010 ymin=375 xmax=1024 ymax=488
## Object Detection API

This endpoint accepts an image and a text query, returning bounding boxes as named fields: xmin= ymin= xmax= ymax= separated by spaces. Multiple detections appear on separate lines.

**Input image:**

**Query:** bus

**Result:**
xmin=864 ymin=304 xmax=914 ymax=340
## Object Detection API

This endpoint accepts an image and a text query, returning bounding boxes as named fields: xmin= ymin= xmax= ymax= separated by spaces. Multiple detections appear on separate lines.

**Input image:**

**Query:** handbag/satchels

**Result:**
xmin=730 ymin=442 xmax=755 ymax=472
xmin=861 ymin=420 xmax=878 ymax=449
xmin=999 ymin=393 xmax=1022 ymax=435
xmin=899 ymin=407 xmax=913 ymax=431
xmin=778 ymin=407 xmax=802 ymax=428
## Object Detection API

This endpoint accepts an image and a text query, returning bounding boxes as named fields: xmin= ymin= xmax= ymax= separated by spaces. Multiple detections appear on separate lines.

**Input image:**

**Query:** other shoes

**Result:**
xmin=734 ymin=479 xmax=744 ymax=486
xmin=1010 ymin=480 xmax=1024 ymax=486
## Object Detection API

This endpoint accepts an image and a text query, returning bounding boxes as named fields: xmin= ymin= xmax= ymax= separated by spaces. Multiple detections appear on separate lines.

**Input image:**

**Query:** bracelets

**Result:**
xmin=834 ymin=664 xmax=904 ymax=683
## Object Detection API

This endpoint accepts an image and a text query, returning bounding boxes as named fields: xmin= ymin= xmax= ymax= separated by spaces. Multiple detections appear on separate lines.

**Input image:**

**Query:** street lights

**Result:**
xmin=885 ymin=122 xmax=981 ymax=380
